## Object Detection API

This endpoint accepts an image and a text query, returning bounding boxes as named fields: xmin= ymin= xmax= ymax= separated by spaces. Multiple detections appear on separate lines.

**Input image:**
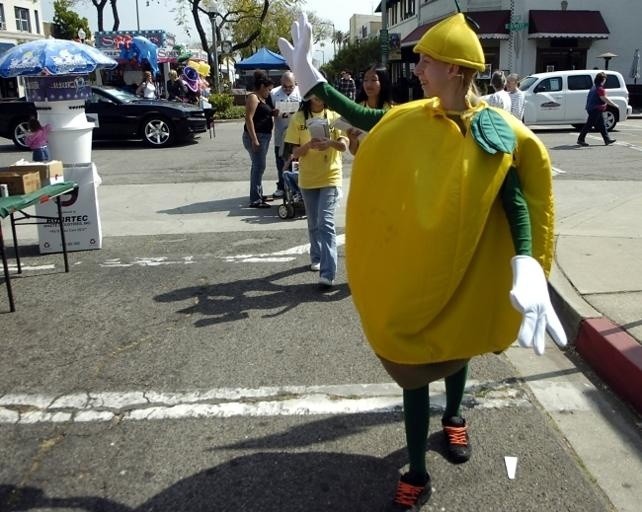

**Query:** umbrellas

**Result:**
xmin=627 ymin=47 xmax=640 ymax=89
xmin=1 ymin=35 xmax=122 ymax=77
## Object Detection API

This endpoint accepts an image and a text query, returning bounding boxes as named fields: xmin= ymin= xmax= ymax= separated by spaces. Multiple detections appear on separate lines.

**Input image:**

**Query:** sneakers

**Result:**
xmin=273 ymin=189 xmax=286 ymax=197
xmin=319 ymin=276 xmax=335 ymax=285
xmin=310 ymin=262 xmax=320 ymax=270
xmin=441 ymin=412 xmax=470 ymax=464
xmin=393 ymin=470 xmax=433 ymax=511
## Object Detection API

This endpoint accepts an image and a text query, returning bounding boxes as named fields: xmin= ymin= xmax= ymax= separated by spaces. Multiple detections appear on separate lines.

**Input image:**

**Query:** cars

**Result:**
xmin=2 ymin=83 xmax=213 ymax=147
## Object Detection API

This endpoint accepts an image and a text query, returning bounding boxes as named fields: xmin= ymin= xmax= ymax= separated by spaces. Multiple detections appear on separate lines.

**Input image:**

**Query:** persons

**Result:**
xmin=272 ymin=7 xmax=572 ymax=512
xmin=343 ymin=60 xmax=398 ymax=156
xmin=268 ymin=72 xmax=302 ymax=198
xmin=339 ymin=72 xmax=357 ymax=104
xmin=136 ymin=71 xmax=156 ymax=100
xmin=284 ymin=74 xmax=353 ymax=293
xmin=489 ymin=68 xmax=512 ymax=112
xmin=505 ymin=74 xmax=527 ymax=122
xmin=240 ymin=72 xmax=273 ymax=211
xmin=575 ymin=71 xmax=620 ymax=147
xmin=165 ymin=70 xmax=190 ymax=103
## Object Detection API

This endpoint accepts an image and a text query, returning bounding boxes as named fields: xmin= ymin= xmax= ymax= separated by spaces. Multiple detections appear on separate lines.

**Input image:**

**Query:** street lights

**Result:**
xmin=317 ymin=20 xmax=337 ymax=59
xmin=76 ymin=27 xmax=87 ymax=44
xmin=315 ymin=48 xmax=324 ymax=65
xmin=221 ymin=26 xmax=232 ymax=84
xmin=595 ymin=50 xmax=619 ymax=69
xmin=206 ymin=0 xmax=223 ymax=97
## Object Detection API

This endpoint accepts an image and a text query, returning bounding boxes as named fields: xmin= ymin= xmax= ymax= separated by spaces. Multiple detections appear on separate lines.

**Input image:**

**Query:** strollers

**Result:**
xmin=278 ymin=150 xmax=305 ymax=219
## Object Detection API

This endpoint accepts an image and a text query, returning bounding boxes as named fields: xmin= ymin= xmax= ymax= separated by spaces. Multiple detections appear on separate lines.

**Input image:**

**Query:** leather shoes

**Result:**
xmin=605 ymin=139 xmax=615 ymax=145
xmin=577 ymin=140 xmax=589 ymax=145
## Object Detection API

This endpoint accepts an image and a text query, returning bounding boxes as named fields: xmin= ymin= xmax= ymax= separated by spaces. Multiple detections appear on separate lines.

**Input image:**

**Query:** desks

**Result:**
xmin=0 ymin=179 xmax=79 ymax=313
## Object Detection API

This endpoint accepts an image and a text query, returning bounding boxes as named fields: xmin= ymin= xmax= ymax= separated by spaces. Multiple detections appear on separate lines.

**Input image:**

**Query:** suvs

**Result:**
xmin=478 ymin=68 xmax=633 ymax=132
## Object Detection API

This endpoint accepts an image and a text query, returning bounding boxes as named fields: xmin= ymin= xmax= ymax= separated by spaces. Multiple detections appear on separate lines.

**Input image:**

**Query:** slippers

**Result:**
xmin=262 ymin=196 xmax=274 ymax=201
xmin=249 ymin=202 xmax=272 ymax=208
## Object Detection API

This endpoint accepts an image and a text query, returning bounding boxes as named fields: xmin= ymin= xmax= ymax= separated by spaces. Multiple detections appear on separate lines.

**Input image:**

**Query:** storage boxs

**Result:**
xmin=0 ymin=161 xmax=65 ymax=185
xmin=0 ymin=169 xmax=41 ymax=195
